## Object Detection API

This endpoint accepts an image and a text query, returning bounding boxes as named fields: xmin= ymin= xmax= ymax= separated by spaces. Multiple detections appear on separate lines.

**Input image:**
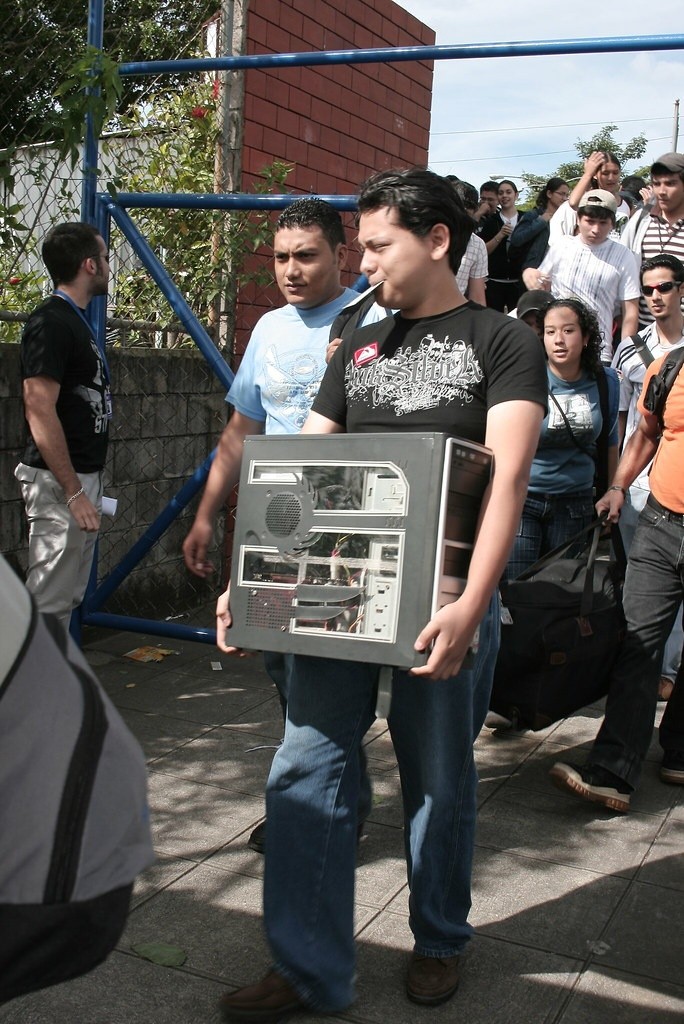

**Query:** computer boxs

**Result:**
xmin=224 ymin=431 xmax=496 ymax=670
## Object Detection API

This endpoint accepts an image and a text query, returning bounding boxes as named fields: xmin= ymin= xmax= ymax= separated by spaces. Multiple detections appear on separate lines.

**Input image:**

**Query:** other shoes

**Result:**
xmin=484 ymin=711 xmax=512 ymax=729
xmin=657 ymin=677 xmax=674 ymax=701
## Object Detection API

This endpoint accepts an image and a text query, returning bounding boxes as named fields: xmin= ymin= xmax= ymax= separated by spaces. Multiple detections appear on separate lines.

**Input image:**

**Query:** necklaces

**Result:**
xmin=657 ymin=219 xmax=677 ymax=253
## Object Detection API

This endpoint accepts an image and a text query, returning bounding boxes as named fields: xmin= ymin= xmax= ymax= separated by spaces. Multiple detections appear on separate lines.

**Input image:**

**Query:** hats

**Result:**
xmin=517 ymin=290 xmax=556 ymax=319
xmin=617 ymin=191 xmax=638 ymax=203
xmin=651 ymin=152 xmax=684 ymax=174
xmin=451 ymin=181 xmax=478 ymax=209
xmin=578 ymin=189 xmax=617 ymax=214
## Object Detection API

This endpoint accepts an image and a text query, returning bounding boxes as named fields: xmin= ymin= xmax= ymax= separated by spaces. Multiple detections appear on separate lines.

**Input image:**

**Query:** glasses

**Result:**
xmin=640 ymin=281 xmax=681 ymax=296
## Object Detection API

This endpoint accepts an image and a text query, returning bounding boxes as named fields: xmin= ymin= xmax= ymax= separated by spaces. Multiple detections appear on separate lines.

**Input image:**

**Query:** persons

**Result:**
xmin=446 ymin=149 xmax=684 ymax=813
xmin=213 ymin=164 xmax=549 ymax=1024
xmin=183 ymin=194 xmax=400 ymax=854
xmin=13 ymin=223 xmax=114 ymax=632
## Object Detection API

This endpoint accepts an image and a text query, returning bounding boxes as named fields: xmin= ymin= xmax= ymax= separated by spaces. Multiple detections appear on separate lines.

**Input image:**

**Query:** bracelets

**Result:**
xmin=495 ymin=236 xmax=500 ymax=243
xmin=607 ymin=486 xmax=626 ymax=500
xmin=66 ymin=487 xmax=85 ymax=507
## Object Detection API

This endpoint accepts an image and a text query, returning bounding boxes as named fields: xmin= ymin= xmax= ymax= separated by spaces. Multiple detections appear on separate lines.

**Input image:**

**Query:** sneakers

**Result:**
xmin=405 ymin=955 xmax=461 ymax=1005
xmin=219 ymin=976 xmax=302 ymax=1021
xmin=660 ymin=751 xmax=684 ymax=784
xmin=549 ymin=761 xmax=634 ymax=813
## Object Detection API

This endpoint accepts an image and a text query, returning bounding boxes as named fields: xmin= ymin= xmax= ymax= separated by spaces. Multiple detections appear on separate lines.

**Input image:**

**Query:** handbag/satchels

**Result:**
xmin=487 ymin=509 xmax=627 ymax=732
